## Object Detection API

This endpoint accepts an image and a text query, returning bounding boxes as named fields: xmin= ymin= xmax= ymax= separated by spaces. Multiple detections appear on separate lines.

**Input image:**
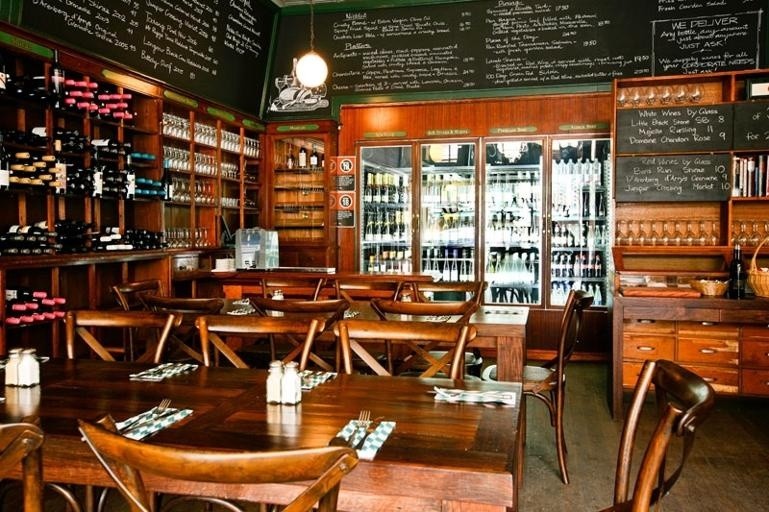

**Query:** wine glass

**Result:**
xmin=271 ymin=153 xmax=324 ymax=214
xmin=274 ymin=77 xmax=285 ymax=94
xmin=615 ymin=85 xmax=705 ymax=108
xmin=613 ymin=210 xmax=768 ymax=249
xmin=159 ymin=111 xmax=260 ymax=247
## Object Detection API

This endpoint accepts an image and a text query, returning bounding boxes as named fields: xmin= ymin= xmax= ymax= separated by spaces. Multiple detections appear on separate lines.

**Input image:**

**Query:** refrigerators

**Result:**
xmin=357 ymin=137 xmax=482 ymax=310
xmin=480 ymin=129 xmax=613 ymax=309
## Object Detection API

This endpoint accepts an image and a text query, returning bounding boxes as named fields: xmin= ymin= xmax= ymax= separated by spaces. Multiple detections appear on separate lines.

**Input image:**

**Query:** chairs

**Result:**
xmin=599 ymin=358 xmax=712 ymax=511
xmin=0 ymin=268 xmax=594 ymax=510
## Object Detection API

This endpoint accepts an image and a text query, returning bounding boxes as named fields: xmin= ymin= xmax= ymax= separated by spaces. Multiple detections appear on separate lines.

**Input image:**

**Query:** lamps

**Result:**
xmin=293 ymin=1 xmax=331 ymax=90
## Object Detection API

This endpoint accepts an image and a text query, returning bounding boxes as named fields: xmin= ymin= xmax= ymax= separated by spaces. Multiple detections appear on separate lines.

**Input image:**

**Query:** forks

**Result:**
xmin=116 ymin=398 xmax=170 ymax=436
xmin=342 ymin=409 xmax=370 ymax=449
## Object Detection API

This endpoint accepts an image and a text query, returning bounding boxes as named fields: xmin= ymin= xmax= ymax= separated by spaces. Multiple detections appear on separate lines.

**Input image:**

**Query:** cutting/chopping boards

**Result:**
xmin=619 ymin=285 xmax=701 ymax=298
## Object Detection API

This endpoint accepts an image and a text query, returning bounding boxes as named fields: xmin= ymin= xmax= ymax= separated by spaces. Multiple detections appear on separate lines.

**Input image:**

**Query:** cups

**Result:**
xmin=216 ymin=258 xmax=235 ymax=269
xmin=283 ymin=74 xmax=296 ymax=88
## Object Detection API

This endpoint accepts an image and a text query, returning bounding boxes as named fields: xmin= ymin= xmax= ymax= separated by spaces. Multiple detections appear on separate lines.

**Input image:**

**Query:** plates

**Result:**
xmin=211 ymin=269 xmax=235 ymax=272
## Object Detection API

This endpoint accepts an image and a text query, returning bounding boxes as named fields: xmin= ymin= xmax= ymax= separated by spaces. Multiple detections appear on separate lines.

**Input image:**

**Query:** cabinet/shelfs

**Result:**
xmin=337 ymin=92 xmax=613 ymax=361
xmin=0 ymin=28 xmax=162 ymax=268
xmin=614 ymin=69 xmax=768 ymax=418
xmin=267 ymin=120 xmax=335 ymax=267
xmin=163 ymin=87 xmax=267 ymax=252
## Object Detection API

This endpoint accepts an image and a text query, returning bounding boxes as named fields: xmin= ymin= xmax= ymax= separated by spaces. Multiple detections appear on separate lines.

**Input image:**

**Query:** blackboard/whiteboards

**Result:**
xmin=0 ymin=0 xmax=278 ymax=120
xmin=270 ymin=0 xmax=769 ymax=94
xmin=612 ymin=99 xmax=769 ymax=202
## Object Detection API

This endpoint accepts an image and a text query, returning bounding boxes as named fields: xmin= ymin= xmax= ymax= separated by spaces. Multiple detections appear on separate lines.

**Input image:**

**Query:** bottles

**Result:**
xmin=298 ymin=138 xmax=307 ymax=170
xmin=264 ymin=401 xmax=281 ymax=445
xmin=0 ymin=44 xmax=170 ymax=256
xmin=271 ymin=290 xmax=279 ymax=318
xmin=277 ymin=290 xmax=285 ymax=318
xmin=281 ymin=403 xmax=303 ymax=447
xmin=1 ymin=384 xmax=20 ymax=425
xmin=321 ymin=145 xmax=324 ymax=170
xmin=4 ymin=280 xmax=68 ymax=326
xmin=17 ymin=386 xmax=40 ymax=424
xmin=265 ymin=359 xmax=283 ymax=405
xmin=289 ymin=58 xmax=301 ymax=90
xmin=14 ymin=348 xmax=42 ymax=388
xmin=727 ymin=243 xmax=746 ymax=301
xmin=281 ymin=361 xmax=301 ymax=407
xmin=400 ymin=294 xmax=407 ymax=302
xmin=406 ymin=294 xmax=411 ymax=303
xmin=2 ymin=347 xmax=21 ymax=387
xmin=284 ymin=141 xmax=294 ymax=170
xmin=308 ymin=141 xmax=319 ymax=170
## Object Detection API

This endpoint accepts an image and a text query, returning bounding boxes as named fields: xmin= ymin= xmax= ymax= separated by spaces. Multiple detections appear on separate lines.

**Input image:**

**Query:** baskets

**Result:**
xmin=746 ymin=234 xmax=769 ymax=298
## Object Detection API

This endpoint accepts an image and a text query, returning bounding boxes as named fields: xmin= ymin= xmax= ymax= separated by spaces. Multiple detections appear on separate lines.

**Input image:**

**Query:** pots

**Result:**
xmin=173 ymin=253 xmax=199 ymax=270
xmin=281 ymin=88 xmax=317 ymax=110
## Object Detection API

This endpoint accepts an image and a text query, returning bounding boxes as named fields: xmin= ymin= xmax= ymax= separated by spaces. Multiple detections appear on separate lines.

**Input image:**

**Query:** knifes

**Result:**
xmin=116 ymin=405 xmax=189 ymax=436
xmin=352 ymin=415 xmax=384 ymax=450
